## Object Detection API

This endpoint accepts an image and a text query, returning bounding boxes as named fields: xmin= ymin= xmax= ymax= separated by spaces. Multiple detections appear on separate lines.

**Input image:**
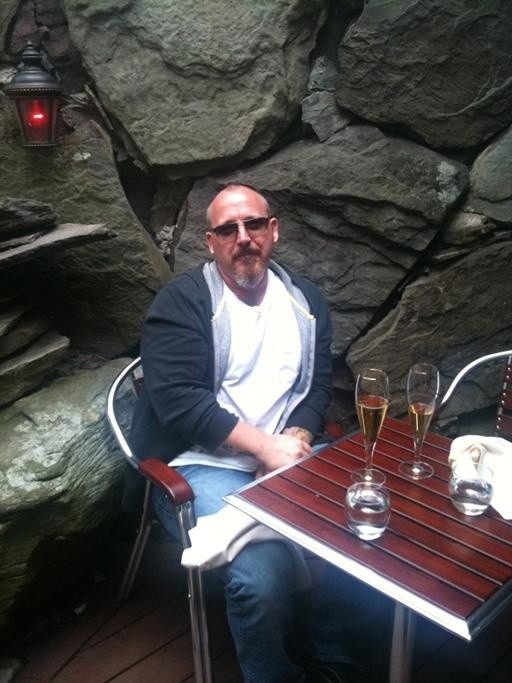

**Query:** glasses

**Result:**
xmin=206 ymin=217 xmax=273 ymax=238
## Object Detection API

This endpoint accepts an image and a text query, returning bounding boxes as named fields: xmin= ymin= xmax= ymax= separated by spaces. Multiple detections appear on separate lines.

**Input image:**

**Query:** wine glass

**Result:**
xmin=403 ymin=366 xmax=437 ymax=481
xmin=350 ymin=362 xmax=387 ymax=490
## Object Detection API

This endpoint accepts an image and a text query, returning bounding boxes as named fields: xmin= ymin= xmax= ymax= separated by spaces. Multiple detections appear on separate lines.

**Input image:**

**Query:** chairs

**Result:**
xmin=426 ymin=344 xmax=511 ymax=447
xmin=100 ymin=351 xmax=350 ymax=682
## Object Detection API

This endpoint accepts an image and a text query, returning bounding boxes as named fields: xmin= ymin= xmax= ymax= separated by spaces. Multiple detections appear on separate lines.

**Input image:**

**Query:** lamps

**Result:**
xmin=2 ymin=33 xmax=66 ymax=153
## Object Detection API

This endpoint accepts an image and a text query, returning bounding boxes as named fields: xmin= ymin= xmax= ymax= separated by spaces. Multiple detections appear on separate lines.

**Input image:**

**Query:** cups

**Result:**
xmin=450 ymin=464 xmax=497 ymax=517
xmin=346 ymin=485 xmax=390 ymax=543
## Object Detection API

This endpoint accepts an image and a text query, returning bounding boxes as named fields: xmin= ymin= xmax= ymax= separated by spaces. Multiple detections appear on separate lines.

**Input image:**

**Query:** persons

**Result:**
xmin=129 ymin=184 xmax=360 ymax=683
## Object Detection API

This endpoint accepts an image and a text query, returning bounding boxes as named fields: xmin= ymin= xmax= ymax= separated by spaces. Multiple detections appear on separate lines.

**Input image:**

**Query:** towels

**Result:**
xmin=444 ymin=430 xmax=511 ymax=525
xmin=180 ymin=494 xmax=313 ymax=601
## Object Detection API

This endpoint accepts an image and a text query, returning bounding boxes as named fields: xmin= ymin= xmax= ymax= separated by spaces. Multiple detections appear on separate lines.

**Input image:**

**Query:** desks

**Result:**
xmin=219 ymin=415 xmax=511 ymax=682
